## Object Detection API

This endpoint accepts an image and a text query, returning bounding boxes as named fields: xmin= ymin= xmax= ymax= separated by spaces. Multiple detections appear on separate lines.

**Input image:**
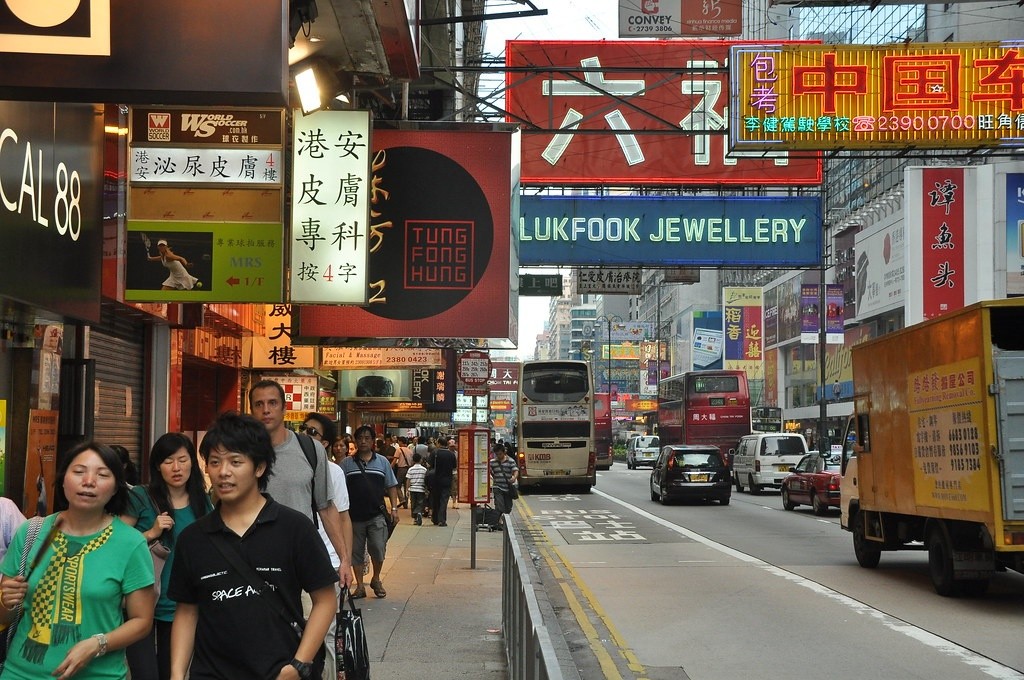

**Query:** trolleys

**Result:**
xmin=476 ymin=485 xmax=510 ymax=532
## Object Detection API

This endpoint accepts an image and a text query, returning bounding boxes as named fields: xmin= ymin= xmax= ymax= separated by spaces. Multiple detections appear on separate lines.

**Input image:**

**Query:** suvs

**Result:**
xmin=650 ymin=444 xmax=732 ymax=505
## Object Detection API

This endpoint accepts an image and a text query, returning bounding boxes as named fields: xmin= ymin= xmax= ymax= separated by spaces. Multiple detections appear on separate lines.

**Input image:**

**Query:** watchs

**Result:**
xmin=290 ymin=657 xmax=316 ymax=679
xmin=93 ymin=633 xmax=107 ymax=658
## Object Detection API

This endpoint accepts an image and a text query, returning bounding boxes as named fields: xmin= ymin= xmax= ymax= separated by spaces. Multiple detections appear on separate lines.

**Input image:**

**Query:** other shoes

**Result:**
xmin=414 ymin=520 xmax=417 ymax=524
xmin=370 ymin=577 xmax=386 ymax=598
xmin=424 ymin=509 xmax=428 ymax=517
xmin=417 ymin=512 xmax=422 ymax=526
xmin=491 ymin=524 xmax=498 ymax=530
xmin=397 ymin=501 xmax=408 ymax=509
xmin=498 ymin=523 xmax=504 ymax=530
xmin=431 ymin=517 xmax=438 ymax=524
xmin=350 ymin=586 xmax=366 ymax=598
xmin=438 ymin=521 xmax=448 ymax=526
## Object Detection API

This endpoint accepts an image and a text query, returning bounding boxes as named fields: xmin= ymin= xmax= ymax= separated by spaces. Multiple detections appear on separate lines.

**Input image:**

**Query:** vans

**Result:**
xmin=729 ymin=432 xmax=809 ymax=495
xmin=626 ymin=435 xmax=660 ymax=469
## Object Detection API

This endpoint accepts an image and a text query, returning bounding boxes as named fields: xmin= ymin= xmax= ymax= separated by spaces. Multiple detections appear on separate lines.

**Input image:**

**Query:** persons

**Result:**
xmin=166 ymin=409 xmax=339 ymax=680
xmin=107 ymin=432 xmax=222 ymax=680
xmin=147 ymin=239 xmax=198 ymax=290
xmin=249 ymin=375 xmax=355 ymax=592
xmin=0 ymin=439 xmax=155 ymax=680
xmin=298 ymin=413 xmax=516 ymax=599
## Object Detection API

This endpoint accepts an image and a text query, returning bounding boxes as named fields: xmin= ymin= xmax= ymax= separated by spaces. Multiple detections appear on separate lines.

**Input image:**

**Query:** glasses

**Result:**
xmin=298 ymin=424 xmax=326 ymax=440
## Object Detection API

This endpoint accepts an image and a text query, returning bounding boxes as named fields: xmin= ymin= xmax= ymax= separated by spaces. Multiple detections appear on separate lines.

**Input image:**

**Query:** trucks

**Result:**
xmin=619 ymin=431 xmax=640 ymax=443
xmin=840 ymin=296 xmax=1024 ymax=598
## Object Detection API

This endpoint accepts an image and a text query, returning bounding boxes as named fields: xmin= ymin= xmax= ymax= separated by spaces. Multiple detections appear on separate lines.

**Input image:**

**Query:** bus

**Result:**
xmin=643 ymin=406 xmax=784 ymax=434
xmin=593 ymin=393 xmax=613 ymax=470
xmin=518 ymin=359 xmax=597 ymax=494
xmin=657 ymin=369 xmax=751 ymax=470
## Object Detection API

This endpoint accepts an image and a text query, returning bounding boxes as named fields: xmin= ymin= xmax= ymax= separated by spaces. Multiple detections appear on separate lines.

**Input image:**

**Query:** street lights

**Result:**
xmin=594 ymin=314 xmax=626 ymax=395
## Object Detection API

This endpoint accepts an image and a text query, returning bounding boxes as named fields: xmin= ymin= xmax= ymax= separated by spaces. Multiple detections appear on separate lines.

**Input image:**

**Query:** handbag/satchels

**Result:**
xmin=509 ymin=484 xmax=519 ymax=500
xmin=385 ymin=510 xmax=397 ymax=538
xmin=119 ymin=485 xmax=171 ymax=612
xmin=334 ymin=585 xmax=371 ymax=680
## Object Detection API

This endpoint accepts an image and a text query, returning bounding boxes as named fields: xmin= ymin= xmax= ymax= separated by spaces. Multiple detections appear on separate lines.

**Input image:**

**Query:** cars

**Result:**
xmin=780 ymin=445 xmax=854 ymax=515
